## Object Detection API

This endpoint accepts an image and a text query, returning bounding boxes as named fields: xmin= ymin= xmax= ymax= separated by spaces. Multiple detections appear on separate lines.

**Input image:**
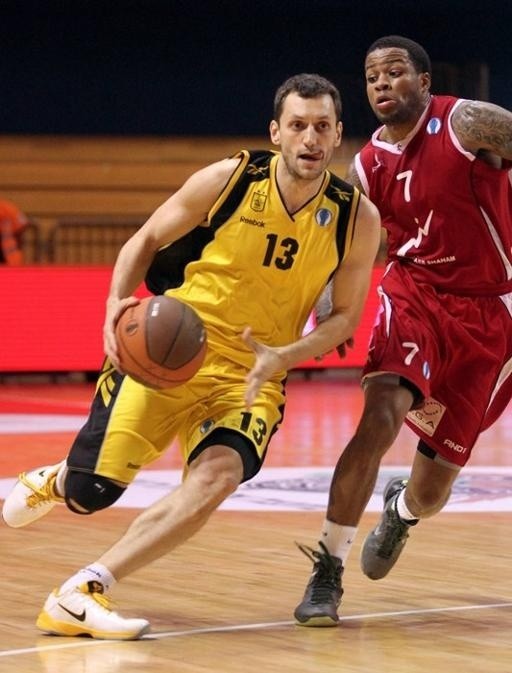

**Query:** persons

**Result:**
xmin=294 ymin=36 xmax=512 ymax=628
xmin=3 ymin=72 xmax=382 ymax=641
xmin=0 ymin=198 xmax=29 ymax=266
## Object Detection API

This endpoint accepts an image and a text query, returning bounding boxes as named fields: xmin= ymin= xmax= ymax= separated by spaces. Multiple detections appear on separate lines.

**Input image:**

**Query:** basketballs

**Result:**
xmin=113 ymin=295 xmax=208 ymax=391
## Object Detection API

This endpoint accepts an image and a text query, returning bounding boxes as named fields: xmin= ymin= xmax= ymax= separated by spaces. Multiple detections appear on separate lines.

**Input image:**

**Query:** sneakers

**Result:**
xmin=359 ymin=478 xmax=420 ymax=579
xmin=2 ymin=458 xmax=65 ymax=528
xmin=35 ymin=580 xmax=151 ymax=640
xmin=295 ymin=541 xmax=344 ymax=628
